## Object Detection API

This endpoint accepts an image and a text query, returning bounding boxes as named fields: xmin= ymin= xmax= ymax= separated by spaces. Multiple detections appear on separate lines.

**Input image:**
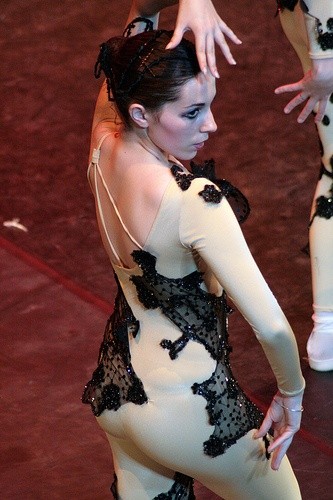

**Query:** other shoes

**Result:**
xmin=306 ymin=305 xmax=333 ymax=371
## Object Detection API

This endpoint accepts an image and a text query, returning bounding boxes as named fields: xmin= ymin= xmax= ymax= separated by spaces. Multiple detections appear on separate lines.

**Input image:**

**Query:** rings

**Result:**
xmin=299 ymin=94 xmax=305 ymax=103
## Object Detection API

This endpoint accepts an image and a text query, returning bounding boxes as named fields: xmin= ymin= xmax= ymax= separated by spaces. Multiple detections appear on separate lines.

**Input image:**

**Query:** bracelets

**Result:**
xmin=271 ymin=397 xmax=304 ymax=417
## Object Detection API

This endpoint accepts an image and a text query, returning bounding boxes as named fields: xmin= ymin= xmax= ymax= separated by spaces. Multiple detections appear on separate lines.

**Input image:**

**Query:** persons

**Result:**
xmin=272 ymin=0 xmax=333 ymax=371
xmin=79 ymin=1 xmax=306 ymax=499
